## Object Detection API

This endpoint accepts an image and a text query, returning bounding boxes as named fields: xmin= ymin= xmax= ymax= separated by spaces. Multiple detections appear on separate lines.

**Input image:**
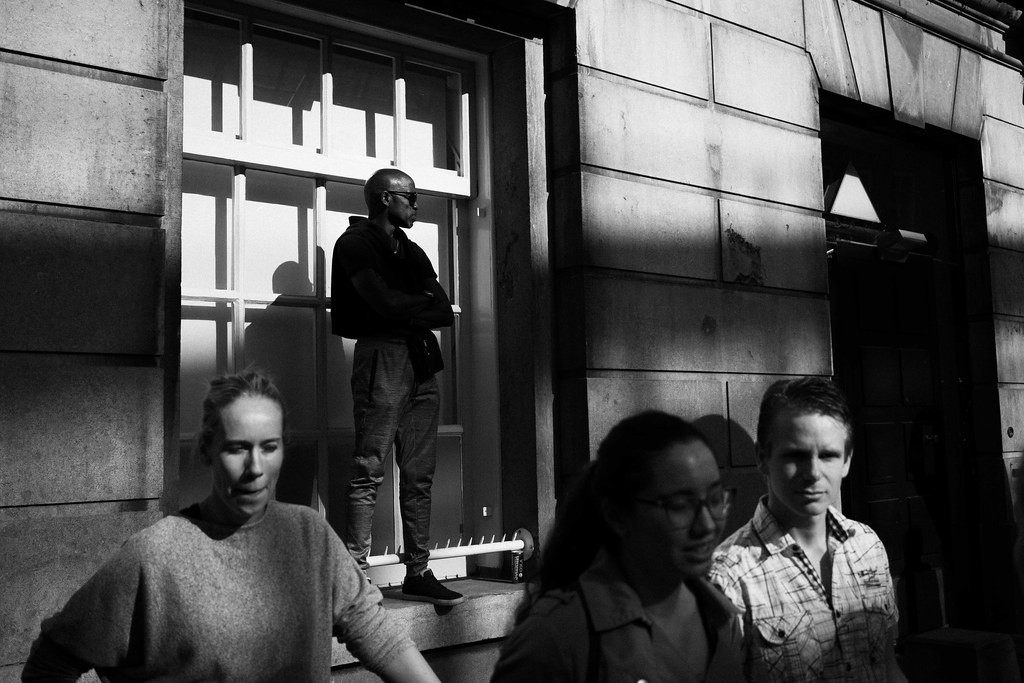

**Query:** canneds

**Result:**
xmin=511 ymin=550 xmax=524 ymax=583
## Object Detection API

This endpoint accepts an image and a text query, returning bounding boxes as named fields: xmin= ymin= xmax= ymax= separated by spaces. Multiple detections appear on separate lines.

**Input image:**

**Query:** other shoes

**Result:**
xmin=401 ymin=568 xmax=465 ymax=607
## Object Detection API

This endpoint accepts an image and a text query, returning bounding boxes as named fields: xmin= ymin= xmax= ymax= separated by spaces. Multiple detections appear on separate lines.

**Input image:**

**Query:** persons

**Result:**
xmin=330 ymin=169 xmax=469 ymax=607
xmin=708 ymin=375 xmax=906 ymax=683
xmin=21 ymin=368 xmax=443 ymax=683
xmin=487 ymin=409 xmax=748 ymax=683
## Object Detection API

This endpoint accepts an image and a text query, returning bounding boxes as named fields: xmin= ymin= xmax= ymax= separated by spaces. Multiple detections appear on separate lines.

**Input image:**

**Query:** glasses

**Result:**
xmin=386 ymin=190 xmax=418 ymax=207
xmin=628 ymin=487 xmax=744 ymax=530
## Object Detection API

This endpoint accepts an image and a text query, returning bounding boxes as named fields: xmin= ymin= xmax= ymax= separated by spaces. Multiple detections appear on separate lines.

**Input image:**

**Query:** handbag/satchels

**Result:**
xmin=411 ymin=329 xmax=444 ymax=380
